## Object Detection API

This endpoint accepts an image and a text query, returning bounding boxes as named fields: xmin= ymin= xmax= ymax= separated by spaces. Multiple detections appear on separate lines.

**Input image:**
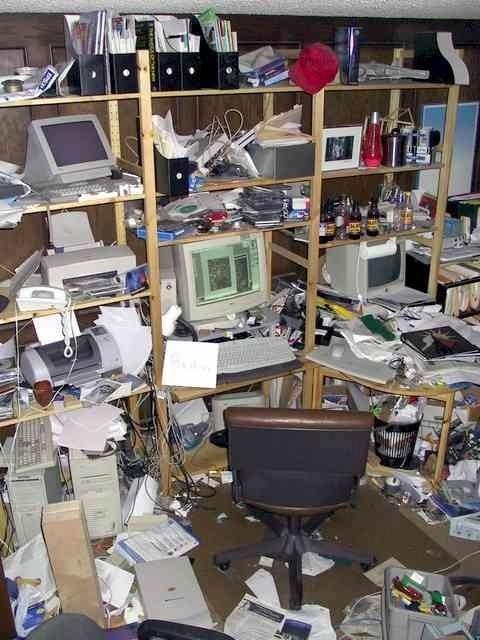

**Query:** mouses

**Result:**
xmin=331 ymin=344 xmax=345 ymax=360
xmin=110 ymin=167 xmax=122 ymax=179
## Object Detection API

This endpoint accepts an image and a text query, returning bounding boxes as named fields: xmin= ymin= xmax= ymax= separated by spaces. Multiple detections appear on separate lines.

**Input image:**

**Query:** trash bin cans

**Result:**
xmin=373 ymin=401 xmax=425 ymax=469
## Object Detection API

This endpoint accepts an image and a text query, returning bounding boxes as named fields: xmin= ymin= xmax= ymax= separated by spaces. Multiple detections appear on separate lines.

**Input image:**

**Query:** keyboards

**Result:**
xmin=13 ymin=416 xmax=57 ymax=474
xmin=218 ymin=335 xmax=297 ymax=375
xmin=44 ymin=182 xmax=121 ymax=203
xmin=305 ymin=346 xmax=397 ymax=384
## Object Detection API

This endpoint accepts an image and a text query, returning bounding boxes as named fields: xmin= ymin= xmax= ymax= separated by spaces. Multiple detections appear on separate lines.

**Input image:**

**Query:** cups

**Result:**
xmin=386 ymin=135 xmax=402 ymax=165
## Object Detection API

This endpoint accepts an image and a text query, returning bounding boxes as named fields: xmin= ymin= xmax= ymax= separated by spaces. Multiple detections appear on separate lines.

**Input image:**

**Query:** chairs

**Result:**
xmin=212 ymin=407 xmax=376 ymax=610
xmin=23 ymin=612 xmax=237 ymax=640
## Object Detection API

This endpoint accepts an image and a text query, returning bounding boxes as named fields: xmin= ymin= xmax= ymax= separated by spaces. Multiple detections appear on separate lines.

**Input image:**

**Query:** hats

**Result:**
xmin=288 ymin=43 xmax=339 ymax=96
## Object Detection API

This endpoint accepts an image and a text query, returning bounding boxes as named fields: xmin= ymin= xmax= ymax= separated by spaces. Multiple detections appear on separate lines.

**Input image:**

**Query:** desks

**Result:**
xmin=406 ymin=232 xmax=480 ymax=319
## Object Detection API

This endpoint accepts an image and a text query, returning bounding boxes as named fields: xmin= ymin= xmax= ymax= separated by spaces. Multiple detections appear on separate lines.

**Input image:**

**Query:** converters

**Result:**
xmin=247 ymin=316 xmax=256 ymax=325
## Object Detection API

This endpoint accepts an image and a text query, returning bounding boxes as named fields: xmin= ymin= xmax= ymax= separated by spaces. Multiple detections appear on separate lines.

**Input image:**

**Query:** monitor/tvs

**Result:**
xmin=174 ymin=231 xmax=270 ymax=332
xmin=23 ymin=113 xmax=125 ymax=188
xmin=325 ymin=239 xmax=407 ymax=299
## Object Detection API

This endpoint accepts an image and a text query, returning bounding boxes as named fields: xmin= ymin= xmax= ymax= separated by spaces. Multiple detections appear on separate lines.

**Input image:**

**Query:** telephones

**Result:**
xmin=15 ymin=285 xmax=69 ymax=312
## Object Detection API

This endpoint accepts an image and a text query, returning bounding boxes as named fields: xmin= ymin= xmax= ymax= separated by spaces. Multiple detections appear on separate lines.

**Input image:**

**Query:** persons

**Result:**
xmin=326 ymin=140 xmax=352 ymax=160
xmin=421 ymin=328 xmax=467 ymax=356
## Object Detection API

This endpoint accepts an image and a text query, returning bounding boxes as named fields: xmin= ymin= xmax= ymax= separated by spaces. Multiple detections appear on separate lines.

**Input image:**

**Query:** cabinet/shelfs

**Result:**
xmin=0 ymin=49 xmax=472 ymax=496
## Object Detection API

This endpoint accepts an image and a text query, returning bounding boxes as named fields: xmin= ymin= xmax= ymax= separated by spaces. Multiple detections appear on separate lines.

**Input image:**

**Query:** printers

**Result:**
xmin=40 ymin=211 xmax=137 ymax=297
xmin=20 ymin=312 xmax=123 ymax=387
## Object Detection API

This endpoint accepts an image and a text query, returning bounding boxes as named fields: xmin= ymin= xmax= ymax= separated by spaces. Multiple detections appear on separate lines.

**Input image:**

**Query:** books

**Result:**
xmin=401 ymin=326 xmax=480 ymax=361
xmin=240 ymin=185 xmax=292 ymax=228
xmin=70 ymin=11 xmax=238 ymax=79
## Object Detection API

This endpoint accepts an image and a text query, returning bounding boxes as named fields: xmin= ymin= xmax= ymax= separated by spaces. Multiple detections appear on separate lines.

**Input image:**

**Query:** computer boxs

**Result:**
xmin=68 ymin=447 xmax=122 ymax=540
xmin=159 ymin=246 xmax=177 ymax=314
xmin=5 ymin=463 xmax=61 ymax=542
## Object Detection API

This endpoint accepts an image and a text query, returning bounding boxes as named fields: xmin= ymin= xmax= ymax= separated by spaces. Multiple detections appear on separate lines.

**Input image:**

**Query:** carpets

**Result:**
xmin=92 ymin=468 xmax=460 ymax=627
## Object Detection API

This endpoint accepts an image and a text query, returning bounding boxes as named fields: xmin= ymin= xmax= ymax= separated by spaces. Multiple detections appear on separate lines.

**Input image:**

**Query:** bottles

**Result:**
xmin=319 ymin=193 xmax=413 ymax=243
xmin=363 ymin=110 xmax=383 ymax=166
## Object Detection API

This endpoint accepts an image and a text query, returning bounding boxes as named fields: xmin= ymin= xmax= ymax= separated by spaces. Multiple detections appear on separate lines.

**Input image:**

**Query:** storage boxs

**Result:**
xmin=382 ymin=565 xmax=461 ymax=640
